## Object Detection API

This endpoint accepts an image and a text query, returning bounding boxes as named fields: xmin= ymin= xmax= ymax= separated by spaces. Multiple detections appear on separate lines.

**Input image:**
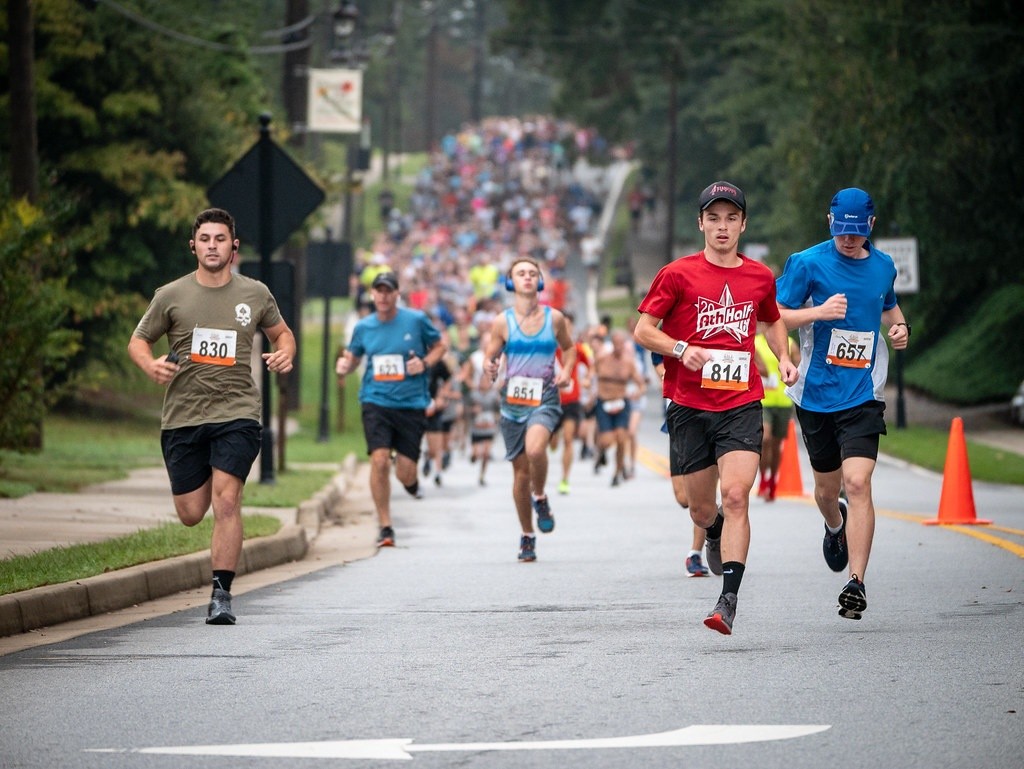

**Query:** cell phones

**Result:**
xmin=165 ymin=351 xmax=179 ymax=364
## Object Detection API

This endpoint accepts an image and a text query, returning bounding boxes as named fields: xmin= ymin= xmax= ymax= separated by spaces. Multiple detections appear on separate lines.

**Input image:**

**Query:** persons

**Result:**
xmin=128 ymin=207 xmax=295 ymax=625
xmin=344 ymin=116 xmax=650 ymax=497
xmin=634 ymin=181 xmax=800 ymax=635
xmin=650 ymin=352 xmax=672 ymax=435
xmin=753 ymin=334 xmax=801 ymax=504
xmin=335 ymin=271 xmax=445 ymax=547
xmin=776 ymin=188 xmax=911 ymax=620
xmin=482 ymin=256 xmax=577 ymax=561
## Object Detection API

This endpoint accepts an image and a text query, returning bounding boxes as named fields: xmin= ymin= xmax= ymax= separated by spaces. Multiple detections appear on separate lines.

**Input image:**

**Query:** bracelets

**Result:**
xmin=423 ymin=361 xmax=430 ymax=374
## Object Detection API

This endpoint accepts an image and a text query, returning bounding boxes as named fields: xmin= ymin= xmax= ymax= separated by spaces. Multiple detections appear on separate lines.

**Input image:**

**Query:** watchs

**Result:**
xmin=672 ymin=341 xmax=689 ymax=358
xmin=897 ymin=323 xmax=911 ymax=337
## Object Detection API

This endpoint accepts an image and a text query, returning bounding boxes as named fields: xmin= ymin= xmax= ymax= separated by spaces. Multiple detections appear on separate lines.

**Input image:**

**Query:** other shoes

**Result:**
xmin=758 ymin=476 xmax=767 ymax=497
xmin=767 ymin=477 xmax=777 ymax=502
xmin=422 ymin=451 xmax=640 ymax=491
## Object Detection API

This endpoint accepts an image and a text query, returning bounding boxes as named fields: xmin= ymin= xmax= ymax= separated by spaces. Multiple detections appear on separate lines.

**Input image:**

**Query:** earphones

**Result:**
xmin=192 ymin=246 xmax=195 ymax=250
xmin=232 ymin=245 xmax=238 ymax=250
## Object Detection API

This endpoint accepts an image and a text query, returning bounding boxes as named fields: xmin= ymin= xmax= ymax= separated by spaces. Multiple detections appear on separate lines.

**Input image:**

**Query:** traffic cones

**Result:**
xmin=774 ymin=416 xmax=808 ymax=498
xmin=922 ymin=415 xmax=998 ymax=528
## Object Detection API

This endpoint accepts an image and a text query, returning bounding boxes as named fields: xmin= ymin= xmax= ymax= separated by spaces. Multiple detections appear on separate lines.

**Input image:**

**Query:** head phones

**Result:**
xmin=504 ymin=267 xmax=544 ymax=293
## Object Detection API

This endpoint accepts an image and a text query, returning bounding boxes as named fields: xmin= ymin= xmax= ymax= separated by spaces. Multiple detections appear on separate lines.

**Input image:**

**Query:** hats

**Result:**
xmin=830 ymin=188 xmax=873 ymax=236
xmin=700 ymin=181 xmax=747 ymax=210
xmin=372 ymin=274 xmax=400 ymax=291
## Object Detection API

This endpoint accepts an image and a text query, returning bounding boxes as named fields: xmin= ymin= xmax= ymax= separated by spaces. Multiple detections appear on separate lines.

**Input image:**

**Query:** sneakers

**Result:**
xmin=823 ymin=497 xmax=849 ymax=573
xmin=404 ymin=480 xmax=418 ymax=495
xmin=706 ymin=505 xmax=725 ymax=576
xmin=704 ymin=593 xmax=738 ymax=635
xmin=518 ymin=537 xmax=537 ymax=562
xmin=205 ymin=590 xmax=236 ymax=624
xmin=685 ymin=555 xmax=709 ymax=576
xmin=839 ymin=574 xmax=867 ymax=620
xmin=377 ymin=527 xmax=395 ymax=546
xmin=533 ymin=495 xmax=555 ymax=533
